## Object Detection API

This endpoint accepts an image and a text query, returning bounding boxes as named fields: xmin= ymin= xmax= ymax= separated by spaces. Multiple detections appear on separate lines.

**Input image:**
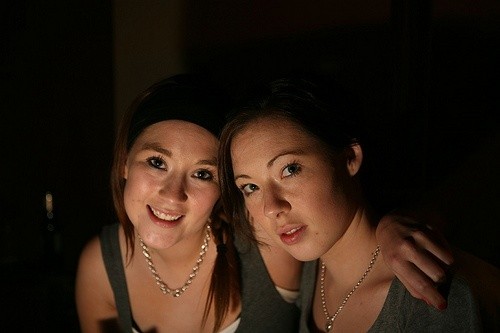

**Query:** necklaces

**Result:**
xmin=321 ymin=247 xmax=380 ymax=331
xmin=136 ymin=221 xmax=212 ymax=300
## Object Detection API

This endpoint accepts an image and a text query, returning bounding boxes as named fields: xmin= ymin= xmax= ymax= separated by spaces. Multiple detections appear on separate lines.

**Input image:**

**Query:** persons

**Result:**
xmin=216 ymin=87 xmax=500 ymax=333
xmin=75 ymin=76 xmax=456 ymax=333
